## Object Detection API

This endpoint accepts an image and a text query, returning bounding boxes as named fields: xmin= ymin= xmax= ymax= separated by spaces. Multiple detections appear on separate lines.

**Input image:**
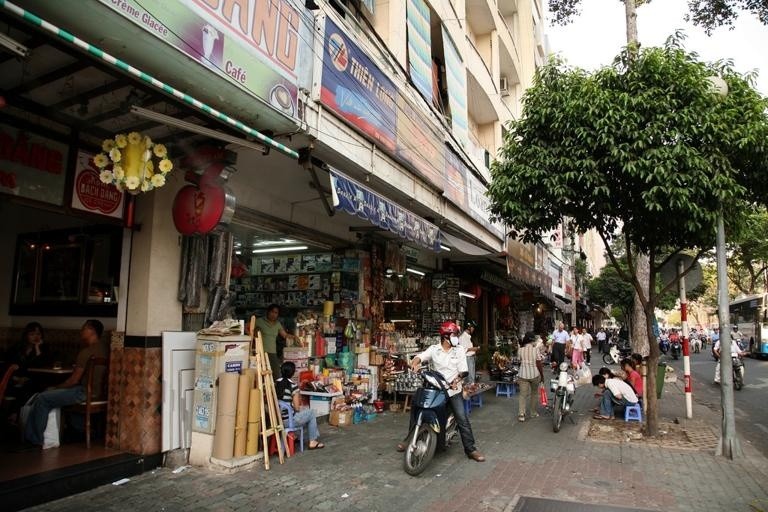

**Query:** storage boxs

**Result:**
xmin=329 ymin=409 xmax=354 ymax=426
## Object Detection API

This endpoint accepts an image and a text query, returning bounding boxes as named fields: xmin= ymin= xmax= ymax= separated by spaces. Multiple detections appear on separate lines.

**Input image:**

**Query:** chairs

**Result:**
xmin=278 ymin=400 xmax=304 ymax=458
xmin=60 ymin=355 xmax=110 ymax=449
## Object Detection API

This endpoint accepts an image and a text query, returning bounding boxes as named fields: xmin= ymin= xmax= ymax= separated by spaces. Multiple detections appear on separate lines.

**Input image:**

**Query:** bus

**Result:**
xmin=713 ymin=291 xmax=768 ymax=361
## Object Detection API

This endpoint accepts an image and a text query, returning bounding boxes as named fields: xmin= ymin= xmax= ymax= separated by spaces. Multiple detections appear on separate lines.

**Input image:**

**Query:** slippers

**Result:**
xmin=308 ymin=442 xmax=325 ymax=449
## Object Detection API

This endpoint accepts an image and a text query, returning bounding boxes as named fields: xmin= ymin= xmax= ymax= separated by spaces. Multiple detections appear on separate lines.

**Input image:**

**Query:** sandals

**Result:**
xmin=468 ymin=450 xmax=485 ymax=462
xmin=589 ymin=406 xmax=617 ymax=421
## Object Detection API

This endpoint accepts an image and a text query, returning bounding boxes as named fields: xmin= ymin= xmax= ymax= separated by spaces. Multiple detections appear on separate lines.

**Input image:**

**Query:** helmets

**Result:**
xmin=439 ymin=321 xmax=461 ymax=337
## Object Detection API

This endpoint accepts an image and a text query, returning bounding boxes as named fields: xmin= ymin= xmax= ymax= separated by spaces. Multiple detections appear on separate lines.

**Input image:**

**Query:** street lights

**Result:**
xmin=698 ymin=71 xmax=753 ymax=459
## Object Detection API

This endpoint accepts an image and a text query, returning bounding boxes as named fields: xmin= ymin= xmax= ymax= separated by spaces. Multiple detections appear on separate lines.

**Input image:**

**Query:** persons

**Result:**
xmin=517 ymin=331 xmax=544 ymax=422
xmin=659 ymin=325 xmax=752 ymax=385
xmin=551 ymin=323 xmax=643 ymax=420
xmin=0 ymin=322 xmax=53 ymax=400
xmin=274 ymin=361 xmax=324 ymax=450
xmin=14 ymin=319 xmax=107 ymax=453
xmin=396 ymin=322 xmax=485 ymax=461
xmin=458 ymin=319 xmax=481 ymax=384
xmin=245 ymin=304 xmax=299 ymax=380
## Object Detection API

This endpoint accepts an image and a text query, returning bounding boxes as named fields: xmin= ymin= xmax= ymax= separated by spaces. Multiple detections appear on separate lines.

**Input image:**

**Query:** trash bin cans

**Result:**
xmin=655 ymin=362 xmax=667 ymax=399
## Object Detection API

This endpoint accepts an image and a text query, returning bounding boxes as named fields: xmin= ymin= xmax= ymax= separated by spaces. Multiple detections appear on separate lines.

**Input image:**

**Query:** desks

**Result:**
xmin=27 ymin=368 xmax=74 ymax=390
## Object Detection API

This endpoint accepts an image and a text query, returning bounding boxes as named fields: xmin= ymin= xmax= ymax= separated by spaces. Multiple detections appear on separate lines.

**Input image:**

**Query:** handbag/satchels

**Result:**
xmin=537 ymin=380 xmax=549 ymax=407
xmin=574 ymin=361 xmax=592 ymax=384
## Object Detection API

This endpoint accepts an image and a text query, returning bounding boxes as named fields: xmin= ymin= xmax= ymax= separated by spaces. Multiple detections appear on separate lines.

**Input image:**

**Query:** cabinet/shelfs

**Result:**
xmin=241 ymin=271 xmax=359 ymax=311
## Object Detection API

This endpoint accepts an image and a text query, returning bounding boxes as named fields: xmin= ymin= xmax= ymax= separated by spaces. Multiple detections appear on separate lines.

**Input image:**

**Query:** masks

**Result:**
xmin=443 ymin=334 xmax=459 ymax=347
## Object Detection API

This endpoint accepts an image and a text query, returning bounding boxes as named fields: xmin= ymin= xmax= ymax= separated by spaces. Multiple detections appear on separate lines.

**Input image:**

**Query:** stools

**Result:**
xmin=624 ymin=404 xmax=642 ymax=423
xmin=471 ymin=393 xmax=485 ymax=408
xmin=495 ymin=383 xmax=513 ymax=399
xmin=463 ymin=400 xmax=472 ymax=417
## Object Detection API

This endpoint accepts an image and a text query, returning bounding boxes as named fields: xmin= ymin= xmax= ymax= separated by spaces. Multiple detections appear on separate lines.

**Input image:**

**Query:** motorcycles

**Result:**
xmin=542 ymin=358 xmax=589 ymax=437
xmin=400 ymin=359 xmax=468 ymax=477
xmin=602 ymin=324 xmax=750 ymax=391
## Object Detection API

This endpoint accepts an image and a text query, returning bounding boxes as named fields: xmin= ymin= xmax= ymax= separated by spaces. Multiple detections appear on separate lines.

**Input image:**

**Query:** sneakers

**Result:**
xmin=586 ymin=362 xmax=591 ymax=366
xmin=517 ymin=412 xmax=540 ymax=422
